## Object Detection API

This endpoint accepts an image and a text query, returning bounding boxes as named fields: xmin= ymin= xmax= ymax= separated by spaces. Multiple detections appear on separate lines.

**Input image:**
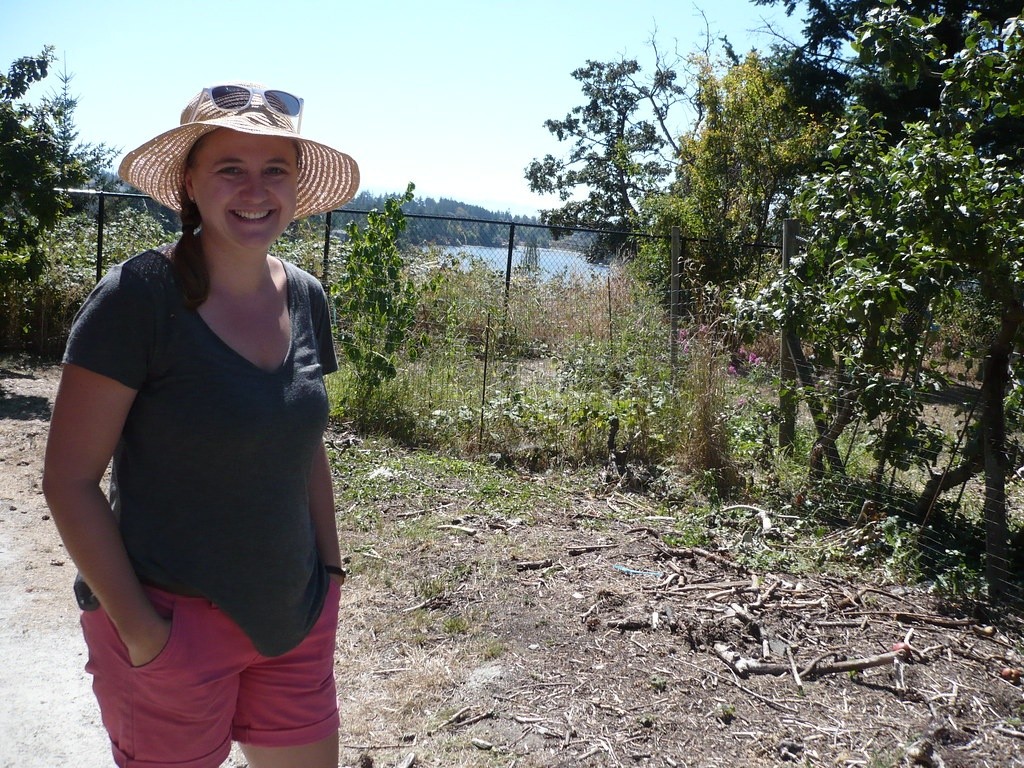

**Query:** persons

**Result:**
xmin=41 ymin=85 xmax=345 ymax=768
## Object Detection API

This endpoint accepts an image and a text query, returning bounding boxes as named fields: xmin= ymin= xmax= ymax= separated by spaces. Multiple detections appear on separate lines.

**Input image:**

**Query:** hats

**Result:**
xmin=119 ymin=83 xmax=359 ymax=220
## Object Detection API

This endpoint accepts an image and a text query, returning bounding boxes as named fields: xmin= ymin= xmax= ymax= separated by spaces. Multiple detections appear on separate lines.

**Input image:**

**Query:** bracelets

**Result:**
xmin=324 ymin=563 xmax=346 ymax=584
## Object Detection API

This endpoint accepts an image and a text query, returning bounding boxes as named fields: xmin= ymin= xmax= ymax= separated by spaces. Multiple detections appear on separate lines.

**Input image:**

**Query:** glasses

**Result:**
xmin=188 ymin=84 xmax=303 ymax=136
